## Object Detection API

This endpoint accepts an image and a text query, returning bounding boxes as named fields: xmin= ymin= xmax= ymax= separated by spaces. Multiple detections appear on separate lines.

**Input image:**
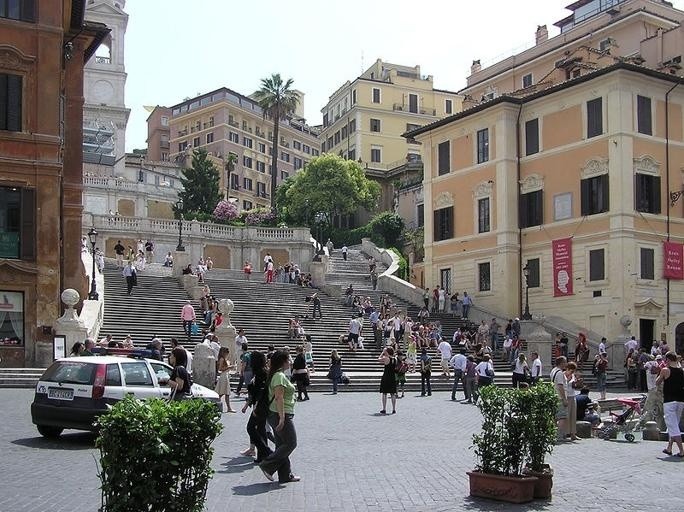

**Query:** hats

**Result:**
xmin=649 ymin=354 xmax=655 ymax=359
xmin=656 ymin=355 xmax=662 ymax=359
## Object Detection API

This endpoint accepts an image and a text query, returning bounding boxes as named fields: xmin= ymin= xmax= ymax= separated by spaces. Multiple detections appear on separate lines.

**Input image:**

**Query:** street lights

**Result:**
xmin=312 ymin=214 xmax=321 ymax=263
xmin=87 ymin=228 xmax=99 ymax=301
xmin=317 ymin=213 xmax=325 ymax=256
xmin=175 ymin=198 xmax=185 ymax=251
xmin=522 ymin=263 xmax=532 ymax=322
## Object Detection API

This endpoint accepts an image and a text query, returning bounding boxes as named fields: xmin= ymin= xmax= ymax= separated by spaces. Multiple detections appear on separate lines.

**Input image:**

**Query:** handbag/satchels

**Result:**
xmin=193 ymin=325 xmax=199 ymax=334
xmin=485 ymin=369 xmax=494 ymax=376
xmin=594 ymin=367 xmax=603 ymax=375
xmin=424 ymin=363 xmax=431 ymax=370
xmin=255 ymin=372 xmax=284 ymax=416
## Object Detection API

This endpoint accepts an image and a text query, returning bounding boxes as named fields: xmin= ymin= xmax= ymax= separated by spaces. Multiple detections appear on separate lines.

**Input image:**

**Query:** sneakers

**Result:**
xmin=239 ymin=449 xmax=256 ymax=456
xmin=290 ymin=475 xmax=300 ymax=481
xmin=262 ymin=468 xmax=274 ymax=481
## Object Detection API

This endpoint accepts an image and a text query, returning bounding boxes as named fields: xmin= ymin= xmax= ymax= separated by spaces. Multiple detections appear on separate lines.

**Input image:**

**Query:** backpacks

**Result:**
xmin=397 ymin=356 xmax=408 ymax=376
xmin=650 ymin=362 xmax=658 ymax=374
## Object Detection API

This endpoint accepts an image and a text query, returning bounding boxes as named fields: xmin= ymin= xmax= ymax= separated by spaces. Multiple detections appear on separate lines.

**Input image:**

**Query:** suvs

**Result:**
xmin=29 ymin=351 xmax=223 ymax=438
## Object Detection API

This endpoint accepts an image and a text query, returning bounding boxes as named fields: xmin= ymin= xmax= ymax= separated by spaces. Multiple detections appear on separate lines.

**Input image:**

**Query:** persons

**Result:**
xmin=400 ymin=285 xmax=541 ymax=403
xmin=259 ymin=350 xmax=301 ymax=483
xmin=325 ymin=239 xmax=377 ymax=289
xmin=241 ymin=351 xmax=270 ymax=457
xmin=550 ymin=332 xmax=669 ymax=443
xmin=306 ymin=282 xmax=400 ymax=413
xmin=114 ymin=240 xmax=144 ymax=296
xmin=71 ymin=334 xmax=192 ymax=378
xmin=653 ymin=350 xmax=684 ymax=458
xmin=181 ymin=295 xmax=315 ymax=413
xmin=244 ymin=252 xmax=312 ymax=287
xmin=156 ymin=349 xmax=194 ymax=402
xmin=145 ymin=240 xmax=212 ymax=296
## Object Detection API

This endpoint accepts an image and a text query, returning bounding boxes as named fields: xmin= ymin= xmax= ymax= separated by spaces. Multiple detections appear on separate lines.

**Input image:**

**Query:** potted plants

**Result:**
xmin=466 ymin=383 xmax=536 ymax=503
xmin=520 ymin=382 xmax=565 ymax=497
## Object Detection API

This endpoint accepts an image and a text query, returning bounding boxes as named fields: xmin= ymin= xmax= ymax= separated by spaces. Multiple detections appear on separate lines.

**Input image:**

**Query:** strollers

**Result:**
xmin=597 ymin=393 xmax=647 ymax=442
